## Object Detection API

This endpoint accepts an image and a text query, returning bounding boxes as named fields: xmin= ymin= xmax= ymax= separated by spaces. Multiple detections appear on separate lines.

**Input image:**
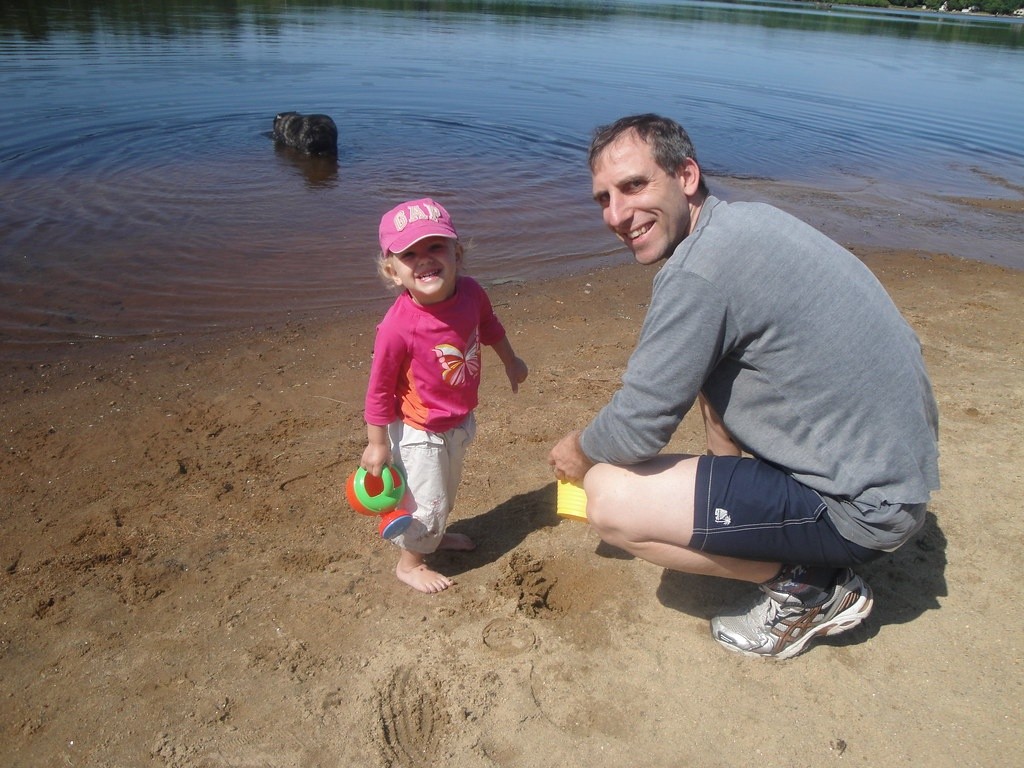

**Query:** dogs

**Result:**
xmin=273 ymin=110 xmax=338 ymax=156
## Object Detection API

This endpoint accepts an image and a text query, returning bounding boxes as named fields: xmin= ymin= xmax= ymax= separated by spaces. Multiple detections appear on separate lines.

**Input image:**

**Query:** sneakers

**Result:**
xmin=710 ymin=566 xmax=874 ymax=662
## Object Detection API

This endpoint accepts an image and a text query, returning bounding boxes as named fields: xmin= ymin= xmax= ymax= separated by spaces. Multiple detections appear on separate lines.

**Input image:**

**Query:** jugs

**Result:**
xmin=347 ymin=464 xmax=414 ymax=541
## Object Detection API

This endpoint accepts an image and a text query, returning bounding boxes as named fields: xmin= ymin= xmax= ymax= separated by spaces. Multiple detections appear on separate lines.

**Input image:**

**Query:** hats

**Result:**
xmin=378 ymin=198 xmax=457 ymax=259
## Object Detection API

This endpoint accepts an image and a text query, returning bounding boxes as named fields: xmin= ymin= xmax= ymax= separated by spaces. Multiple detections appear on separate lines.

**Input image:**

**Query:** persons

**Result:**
xmin=547 ymin=110 xmax=943 ymax=662
xmin=359 ymin=198 xmax=530 ymax=593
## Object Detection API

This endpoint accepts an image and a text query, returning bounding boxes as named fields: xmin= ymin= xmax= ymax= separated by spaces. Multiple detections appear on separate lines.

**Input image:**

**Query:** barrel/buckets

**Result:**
xmin=556 ymin=477 xmax=590 ymax=524
